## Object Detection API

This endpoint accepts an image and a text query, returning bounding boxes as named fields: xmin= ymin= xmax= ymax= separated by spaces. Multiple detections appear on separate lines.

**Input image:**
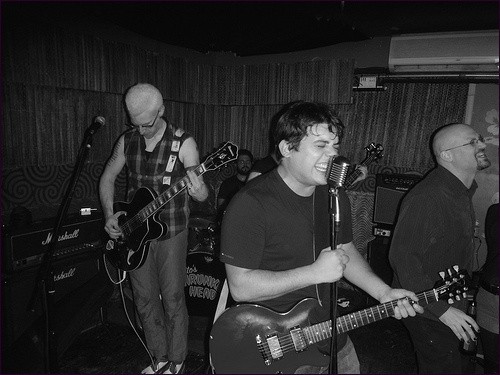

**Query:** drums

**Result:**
xmin=209 ymin=265 xmax=470 ymax=375
xmin=184 ymin=236 xmax=243 ymax=317
xmin=187 ymin=217 xmax=219 ymax=249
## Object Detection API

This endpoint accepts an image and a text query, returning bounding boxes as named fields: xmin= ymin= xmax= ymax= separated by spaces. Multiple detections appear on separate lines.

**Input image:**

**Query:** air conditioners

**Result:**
xmin=388 ymin=30 xmax=499 ymax=74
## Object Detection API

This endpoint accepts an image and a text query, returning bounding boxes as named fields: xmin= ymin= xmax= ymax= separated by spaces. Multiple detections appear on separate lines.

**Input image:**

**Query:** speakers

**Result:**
xmin=368 ymin=227 xmax=395 ymax=288
xmin=374 ymin=185 xmax=406 ymax=225
xmin=6 ymin=232 xmax=112 ymax=375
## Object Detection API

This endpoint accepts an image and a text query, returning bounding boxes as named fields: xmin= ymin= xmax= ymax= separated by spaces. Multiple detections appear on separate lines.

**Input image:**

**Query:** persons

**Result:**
xmin=99 ymin=83 xmax=209 ymax=374
xmin=219 ymin=101 xmax=424 ymax=374
xmin=218 ymin=150 xmax=253 ymax=212
xmin=389 ymin=123 xmax=488 ymax=374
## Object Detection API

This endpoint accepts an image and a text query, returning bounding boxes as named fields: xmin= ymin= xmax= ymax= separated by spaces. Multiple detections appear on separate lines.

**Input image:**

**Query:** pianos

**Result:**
xmin=0 ymin=209 xmax=111 ymax=358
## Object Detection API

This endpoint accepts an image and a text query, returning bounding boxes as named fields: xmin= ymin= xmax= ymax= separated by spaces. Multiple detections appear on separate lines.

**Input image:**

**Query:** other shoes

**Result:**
xmin=141 ymin=360 xmax=186 ymax=375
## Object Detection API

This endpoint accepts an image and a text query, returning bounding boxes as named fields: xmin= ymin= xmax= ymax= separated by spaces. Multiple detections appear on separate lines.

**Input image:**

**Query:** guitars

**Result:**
xmin=101 ymin=140 xmax=238 ymax=270
xmin=343 ymin=142 xmax=384 ymax=192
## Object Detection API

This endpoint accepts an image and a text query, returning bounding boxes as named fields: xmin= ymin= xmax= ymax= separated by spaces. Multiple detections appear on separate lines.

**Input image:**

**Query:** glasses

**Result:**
xmin=237 ymin=160 xmax=252 ymax=165
xmin=125 ymin=110 xmax=160 ymax=129
xmin=444 ymin=136 xmax=485 ymax=151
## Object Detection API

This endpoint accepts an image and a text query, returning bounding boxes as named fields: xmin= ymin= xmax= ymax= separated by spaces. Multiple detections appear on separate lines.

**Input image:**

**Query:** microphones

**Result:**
xmin=84 ymin=115 xmax=106 ymax=140
xmin=328 ymin=155 xmax=351 ymax=191
